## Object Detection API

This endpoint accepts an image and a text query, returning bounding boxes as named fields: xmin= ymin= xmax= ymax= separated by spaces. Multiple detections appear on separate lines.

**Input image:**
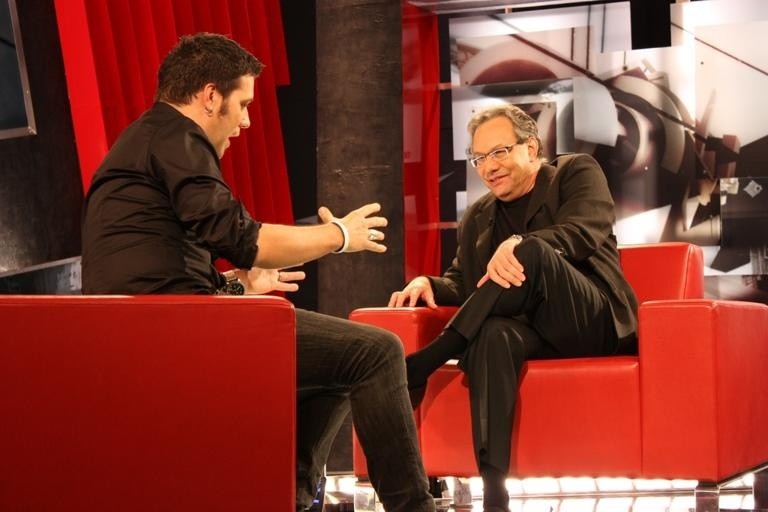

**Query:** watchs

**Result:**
xmin=222 ymin=268 xmax=245 ymax=297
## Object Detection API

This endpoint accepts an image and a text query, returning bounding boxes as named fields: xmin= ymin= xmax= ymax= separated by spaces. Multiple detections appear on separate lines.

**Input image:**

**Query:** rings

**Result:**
xmin=369 ymin=230 xmax=377 ymax=241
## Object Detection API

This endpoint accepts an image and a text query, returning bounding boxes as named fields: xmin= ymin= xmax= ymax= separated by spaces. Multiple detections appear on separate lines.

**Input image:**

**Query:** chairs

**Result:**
xmin=346 ymin=241 xmax=768 ymax=511
xmin=0 ymin=292 xmax=299 ymax=511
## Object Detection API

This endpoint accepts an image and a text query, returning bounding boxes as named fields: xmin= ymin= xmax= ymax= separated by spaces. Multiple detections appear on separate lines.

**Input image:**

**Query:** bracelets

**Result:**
xmin=510 ymin=234 xmax=523 ymax=242
xmin=328 ymin=220 xmax=349 ymax=254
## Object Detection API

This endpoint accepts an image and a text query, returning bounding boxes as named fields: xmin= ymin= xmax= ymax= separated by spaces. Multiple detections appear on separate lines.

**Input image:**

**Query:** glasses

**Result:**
xmin=469 ymin=139 xmax=526 ymax=169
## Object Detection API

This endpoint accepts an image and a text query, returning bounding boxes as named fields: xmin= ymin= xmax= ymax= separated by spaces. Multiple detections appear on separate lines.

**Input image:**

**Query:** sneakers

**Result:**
xmin=407 ymin=381 xmax=428 ymax=411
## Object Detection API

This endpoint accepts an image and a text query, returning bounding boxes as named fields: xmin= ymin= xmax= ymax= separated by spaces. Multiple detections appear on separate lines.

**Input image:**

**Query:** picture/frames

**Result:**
xmin=1 ymin=0 xmax=37 ymax=140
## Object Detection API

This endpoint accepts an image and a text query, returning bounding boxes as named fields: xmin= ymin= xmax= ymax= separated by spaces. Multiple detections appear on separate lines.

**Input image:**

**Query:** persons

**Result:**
xmin=80 ymin=31 xmax=436 ymax=512
xmin=385 ymin=106 xmax=639 ymax=512
